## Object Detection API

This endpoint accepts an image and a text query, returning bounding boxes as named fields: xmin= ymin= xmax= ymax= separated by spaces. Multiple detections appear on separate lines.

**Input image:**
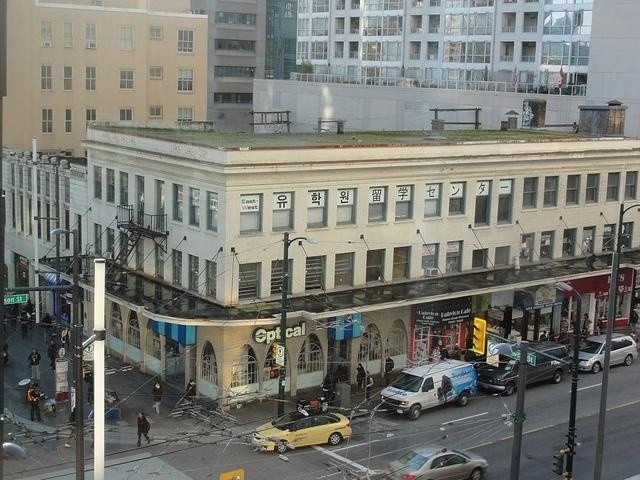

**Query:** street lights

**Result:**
xmin=50 ymin=228 xmax=80 ymax=422
xmin=613 ymin=204 xmax=640 ymax=331
xmin=278 ymin=232 xmax=318 ymax=417
xmin=556 ymin=281 xmax=581 ymax=480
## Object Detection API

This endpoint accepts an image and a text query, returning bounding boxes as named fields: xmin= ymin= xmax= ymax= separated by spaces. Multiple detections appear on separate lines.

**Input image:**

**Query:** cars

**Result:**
xmin=386 ymin=445 xmax=489 ymax=480
xmin=475 ymin=342 xmax=570 ymax=395
xmin=379 ymin=359 xmax=478 ymax=420
xmin=569 ymin=332 xmax=638 ymax=373
xmin=252 ymin=409 xmax=352 ymax=453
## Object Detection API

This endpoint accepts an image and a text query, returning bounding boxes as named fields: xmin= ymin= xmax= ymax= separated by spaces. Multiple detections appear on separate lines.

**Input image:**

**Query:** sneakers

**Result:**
xmin=136 ymin=444 xmax=142 ymax=447
xmin=147 ymin=439 xmax=150 ymax=444
xmin=65 ymin=443 xmax=71 ymax=447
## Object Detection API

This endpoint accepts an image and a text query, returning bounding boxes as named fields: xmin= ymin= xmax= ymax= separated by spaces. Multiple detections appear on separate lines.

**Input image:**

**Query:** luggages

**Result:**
xmin=351 ymin=382 xmax=359 ymax=393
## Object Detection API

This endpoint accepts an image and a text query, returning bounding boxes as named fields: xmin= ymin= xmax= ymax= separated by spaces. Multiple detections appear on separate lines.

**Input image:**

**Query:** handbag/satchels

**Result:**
xmin=320 ymin=382 xmax=324 ymax=387
xmin=29 ymin=363 xmax=32 ymax=368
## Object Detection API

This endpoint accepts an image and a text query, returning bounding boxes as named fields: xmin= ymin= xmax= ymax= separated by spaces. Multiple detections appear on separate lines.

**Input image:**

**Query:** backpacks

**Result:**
xmin=146 ymin=421 xmax=151 ymax=433
xmin=69 ymin=416 xmax=73 ymax=425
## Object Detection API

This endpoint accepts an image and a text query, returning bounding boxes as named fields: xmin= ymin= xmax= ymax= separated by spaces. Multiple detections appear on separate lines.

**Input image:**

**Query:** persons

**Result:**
xmin=323 ymin=374 xmax=331 ymax=400
xmin=385 ymin=356 xmax=393 ymax=373
xmin=137 ymin=413 xmax=150 ymax=447
xmin=362 ymin=371 xmax=373 ymax=398
xmin=583 ymin=314 xmax=592 ymax=334
xmin=28 ymin=383 xmax=44 ymax=422
xmin=539 ymin=331 xmax=549 ymax=343
xmin=61 ymin=324 xmax=72 ymax=350
xmin=28 ymin=348 xmax=41 ymax=380
xmin=11 ymin=299 xmax=33 ymax=339
xmin=47 ymin=339 xmax=59 ymax=369
xmin=152 ymin=381 xmax=162 ymax=413
xmin=357 ymin=363 xmax=365 ymax=388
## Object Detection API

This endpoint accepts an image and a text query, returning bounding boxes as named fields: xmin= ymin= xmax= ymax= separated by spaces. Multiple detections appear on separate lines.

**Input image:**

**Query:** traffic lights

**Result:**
xmin=472 ymin=321 xmax=483 ymax=349
xmin=553 ymin=455 xmax=561 ymax=474
